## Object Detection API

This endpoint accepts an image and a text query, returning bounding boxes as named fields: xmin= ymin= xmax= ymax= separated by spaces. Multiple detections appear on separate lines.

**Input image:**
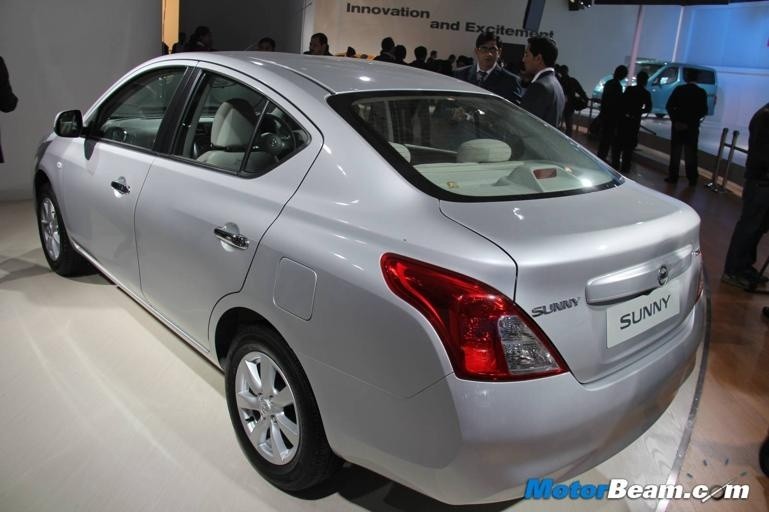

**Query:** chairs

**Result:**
xmin=195 ymin=98 xmax=277 ymax=173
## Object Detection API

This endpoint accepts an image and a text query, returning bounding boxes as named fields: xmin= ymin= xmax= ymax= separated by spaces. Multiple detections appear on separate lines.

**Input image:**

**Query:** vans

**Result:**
xmin=590 ymin=54 xmax=719 ymax=124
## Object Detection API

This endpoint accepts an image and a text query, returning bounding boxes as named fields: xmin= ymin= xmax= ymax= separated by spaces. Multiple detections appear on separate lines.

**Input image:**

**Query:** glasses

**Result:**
xmin=477 ymin=45 xmax=500 ymax=54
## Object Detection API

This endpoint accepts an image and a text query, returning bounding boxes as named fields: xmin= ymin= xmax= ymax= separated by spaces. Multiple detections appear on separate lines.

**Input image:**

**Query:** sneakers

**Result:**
xmin=663 ymin=177 xmax=698 ymax=186
xmin=721 ymin=265 xmax=769 ymax=293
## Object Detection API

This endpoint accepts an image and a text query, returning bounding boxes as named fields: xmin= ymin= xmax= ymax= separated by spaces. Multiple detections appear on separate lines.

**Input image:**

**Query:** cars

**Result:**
xmin=27 ymin=48 xmax=715 ymax=509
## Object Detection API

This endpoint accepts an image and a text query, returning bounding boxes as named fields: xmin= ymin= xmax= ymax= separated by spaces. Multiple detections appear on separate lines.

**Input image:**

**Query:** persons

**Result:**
xmin=257 ymin=37 xmax=276 ymax=52
xmin=718 ymin=101 xmax=768 ymax=291
xmin=663 ymin=69 xmax=709 ymax=187
xmin=373 ymin=37 xmax=398 ymax=64
xmin=503 ymin=34 xmax=566 ymax=160
xmin=455 ymin=55 xmax=466 ymax=67
xmin=502 ymin=58 xmax=521 ymax=77
xmin=444 ymin=30 xmax=521 ymax=153
xmin=1 ymin=54 xmax=20 ymax=166
xmin=302 ymin=32 xmax=334 ymax=56
xmin=162 ymin=41 xmax=170 ymax=55
xmin=345 ymin=46 xmax=356 ymax=57
xmin=408 ymin=45 xmax=443 ymax=150
xmin=591 ymin=64 xmax=628 ymax=161
xmin=610 ymin=69 xmax=653 ymax=174
xmin=447 ymin=54 xmax=458 ymax=62
xmin=171 ymin=31 xmax=188 ymax=53
xmin=558 ymin=64 xmax=589 ymax=137
xmin=425 ymin=50 xmax=437 ymax=65
xmin=184 ymin=25 xmax=218 ymax=51
xmin=395 ymin=44 xmax=416 ymax=149
